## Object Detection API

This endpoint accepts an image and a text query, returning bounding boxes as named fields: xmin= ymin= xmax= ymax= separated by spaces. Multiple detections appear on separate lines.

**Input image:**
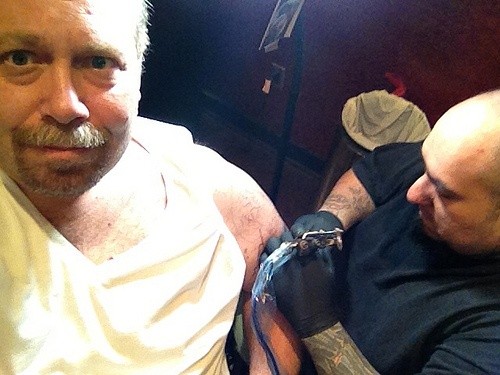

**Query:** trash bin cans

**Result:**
xmin=316 ymin=87 xmax=432 ymax=214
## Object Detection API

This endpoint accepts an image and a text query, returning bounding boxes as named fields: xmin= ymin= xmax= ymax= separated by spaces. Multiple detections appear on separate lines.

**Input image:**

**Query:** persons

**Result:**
xmin=0 ymin=0 xmax=302 ymax=375
xmin=258 ymin=89 xmax=500 ymax=375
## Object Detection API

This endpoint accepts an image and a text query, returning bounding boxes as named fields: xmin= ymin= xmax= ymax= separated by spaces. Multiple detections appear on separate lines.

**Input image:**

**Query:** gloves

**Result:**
xmin=292 ymin=211 xmax=344 ymax=253
xmin=257 ymin=226 xmax=339 ymax=338
